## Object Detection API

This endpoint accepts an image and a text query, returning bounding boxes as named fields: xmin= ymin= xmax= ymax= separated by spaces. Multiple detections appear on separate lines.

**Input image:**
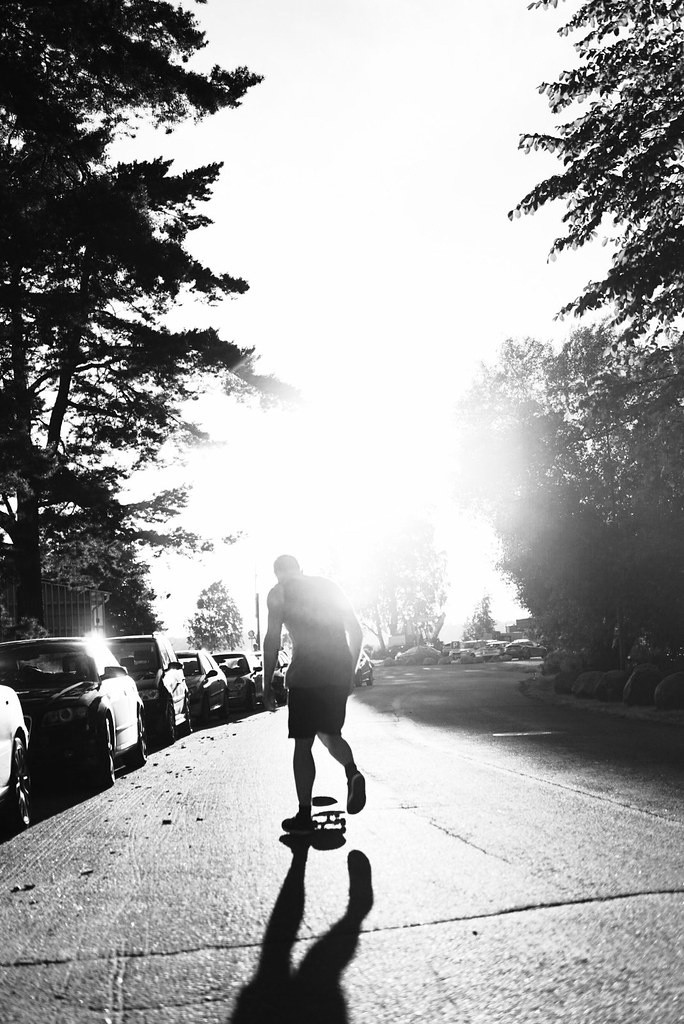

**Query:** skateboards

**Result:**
xmin=311 ymin=796 xmax=346 ymax=832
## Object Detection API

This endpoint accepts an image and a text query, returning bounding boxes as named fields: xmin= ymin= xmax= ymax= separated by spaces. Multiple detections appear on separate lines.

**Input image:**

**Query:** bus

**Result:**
xmin=459 ymin=640 xmax=498 ymax=659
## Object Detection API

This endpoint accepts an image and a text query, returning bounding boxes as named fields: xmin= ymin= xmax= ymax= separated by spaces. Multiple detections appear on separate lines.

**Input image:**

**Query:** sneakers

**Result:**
xmin=282 ymin=812 xmax=314 ymax=833
xmin=347 ymin=771 xmax=367 ymax=815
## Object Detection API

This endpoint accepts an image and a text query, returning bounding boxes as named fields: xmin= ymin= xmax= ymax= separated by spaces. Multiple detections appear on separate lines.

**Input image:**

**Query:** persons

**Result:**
xmin=262 ymin=556 xmax=367 ymax=832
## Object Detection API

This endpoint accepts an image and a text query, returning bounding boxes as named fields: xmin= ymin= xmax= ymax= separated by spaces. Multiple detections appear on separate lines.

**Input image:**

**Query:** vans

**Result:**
xmin=482 ymin=641 xmax=510 ymax=659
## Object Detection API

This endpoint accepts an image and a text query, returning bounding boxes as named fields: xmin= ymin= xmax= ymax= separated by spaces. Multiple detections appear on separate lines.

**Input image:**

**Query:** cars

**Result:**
xmin=443 ymin=643 xmax=450 ymax=654
xmin=0 ymin=684 xmax=36 ymax=829
xmin=253 ymin=650 xmax=292 ymax=707
xmin=353 ymin=652 xmax=374 ymax=687
xmin=102 ymin=635 xmax=194 ymax=746
xmin=174 ymin=649 xmax=231 ymax=721
xmin=212 ymin=651 xmax=261 ymax=709
xmin=505 ymin=639 xmax=547 ymax=661
xmin=448 ymin=643 xmax=460 ymax=660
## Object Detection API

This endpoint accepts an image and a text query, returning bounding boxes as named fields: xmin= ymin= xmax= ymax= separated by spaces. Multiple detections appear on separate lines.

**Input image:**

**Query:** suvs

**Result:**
xmin=0 ymin=636 xmax=148 ymax=788
